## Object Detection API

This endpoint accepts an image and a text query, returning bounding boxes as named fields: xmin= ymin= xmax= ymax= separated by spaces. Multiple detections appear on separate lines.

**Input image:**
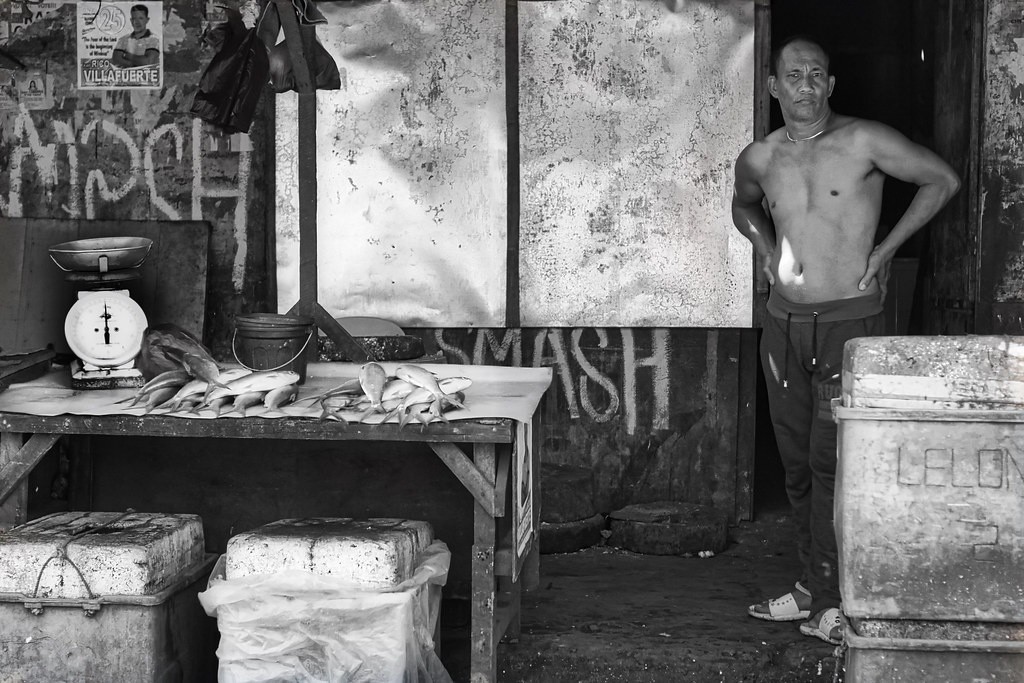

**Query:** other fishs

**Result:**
xmin=115 ymin=347 xmax=476 ymax=435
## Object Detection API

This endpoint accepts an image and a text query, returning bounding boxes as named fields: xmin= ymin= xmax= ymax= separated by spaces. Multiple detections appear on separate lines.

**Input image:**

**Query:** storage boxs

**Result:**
xmin=0 ymin=511 xmax=217 ymax=683
xmin=831 ymin=334 xmax=1024 ymax=683
xmin=197 ymin=517 xmax=451 ymax=683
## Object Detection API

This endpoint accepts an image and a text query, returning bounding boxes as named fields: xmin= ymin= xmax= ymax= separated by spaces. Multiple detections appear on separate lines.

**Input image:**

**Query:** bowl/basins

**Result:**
xmin=235 ymin=312 xmax=315 ymax=329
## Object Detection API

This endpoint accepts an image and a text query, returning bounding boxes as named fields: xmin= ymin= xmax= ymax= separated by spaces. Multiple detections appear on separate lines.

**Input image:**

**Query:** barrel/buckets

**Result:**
xmin=236 ymin=330 xmax=309 ymax=385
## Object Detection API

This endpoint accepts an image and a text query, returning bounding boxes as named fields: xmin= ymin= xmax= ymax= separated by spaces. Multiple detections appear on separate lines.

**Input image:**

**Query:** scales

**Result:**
xmin=48 ymin=236 xmax=154 ymax=390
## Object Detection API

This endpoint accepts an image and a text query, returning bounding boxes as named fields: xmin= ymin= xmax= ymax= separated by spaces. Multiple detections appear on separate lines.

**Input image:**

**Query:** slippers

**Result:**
xmin=747 ymin=591 xmax=813 ymax=621
xmin=800 ymin=606 xmax=843 ymax=645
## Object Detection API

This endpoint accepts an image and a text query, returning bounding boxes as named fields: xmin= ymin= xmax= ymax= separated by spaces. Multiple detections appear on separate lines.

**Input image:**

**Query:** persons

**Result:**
xmin=732 ymin=35 xmax=961 ymax=646
xmin=113 ymin=5 xmax=160 ymax=86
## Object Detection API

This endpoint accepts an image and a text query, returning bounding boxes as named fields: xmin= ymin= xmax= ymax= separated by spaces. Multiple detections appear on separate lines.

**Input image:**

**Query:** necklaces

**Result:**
xmin=786 ymin=130 xmax=826 ymax=141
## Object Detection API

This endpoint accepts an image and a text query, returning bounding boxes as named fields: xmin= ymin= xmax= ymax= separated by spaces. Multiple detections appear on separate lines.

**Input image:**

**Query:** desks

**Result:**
xmin=0 ymin=361 xmax=553 ymax=683
